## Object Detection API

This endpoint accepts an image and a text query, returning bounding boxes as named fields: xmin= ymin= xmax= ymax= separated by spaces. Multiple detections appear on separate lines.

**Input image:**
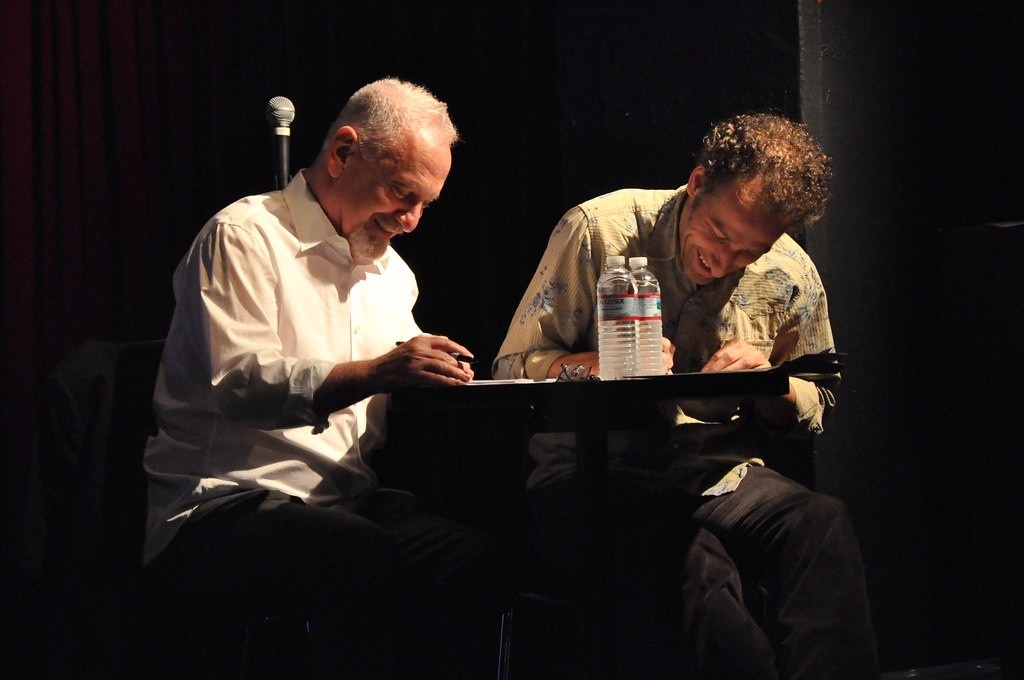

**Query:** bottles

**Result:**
xmin=597 ymin=256 xmax=637 ymax=380
xmin=629 ymin=257 xmax=664 ymax=375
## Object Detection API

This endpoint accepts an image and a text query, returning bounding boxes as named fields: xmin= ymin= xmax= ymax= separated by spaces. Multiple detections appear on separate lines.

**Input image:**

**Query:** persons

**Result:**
xmin=142 ymin=77 xmax=514 ymax=680
xmin=491 ymin=110 xmax=882 ymax=680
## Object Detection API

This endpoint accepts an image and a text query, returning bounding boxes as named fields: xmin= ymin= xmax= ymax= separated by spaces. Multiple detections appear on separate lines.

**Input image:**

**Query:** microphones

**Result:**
xmin=266 ymin=96 xmax=295 ymax=190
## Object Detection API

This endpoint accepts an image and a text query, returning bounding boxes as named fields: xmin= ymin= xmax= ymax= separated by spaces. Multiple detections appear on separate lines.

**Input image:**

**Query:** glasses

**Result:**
xmin=553 ymin=363 xmax=592 ymax=382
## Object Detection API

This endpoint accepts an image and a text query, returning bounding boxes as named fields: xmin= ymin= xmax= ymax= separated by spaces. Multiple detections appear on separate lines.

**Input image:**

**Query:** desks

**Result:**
xmin=392 ymin=363 xmax=793 ymax=679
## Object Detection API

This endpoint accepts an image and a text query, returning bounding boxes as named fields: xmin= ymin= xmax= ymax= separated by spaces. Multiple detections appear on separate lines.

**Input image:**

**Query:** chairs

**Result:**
xmin=487 ymin=408 xmax=818 ymax=679
xmin=96 ymin=339 xmax=424 ymax=680
